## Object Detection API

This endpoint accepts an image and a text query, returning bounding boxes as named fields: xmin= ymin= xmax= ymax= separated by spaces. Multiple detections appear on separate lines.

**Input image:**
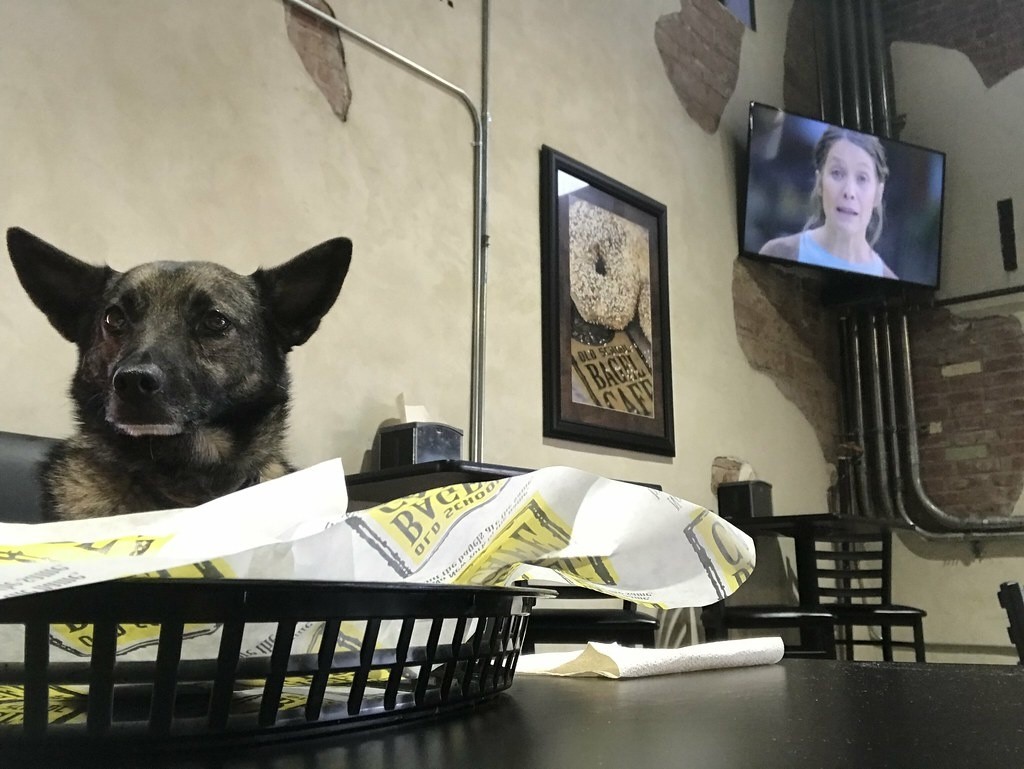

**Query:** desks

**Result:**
xmin=722 ymin=512 xmax=915 ymax=651
xmin=373 ymin=459 xmax=662 ymax=509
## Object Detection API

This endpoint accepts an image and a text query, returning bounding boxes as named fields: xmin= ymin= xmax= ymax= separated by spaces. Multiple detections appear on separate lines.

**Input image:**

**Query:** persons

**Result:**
xmin=758 ymin=124 xmax=900 ymax=280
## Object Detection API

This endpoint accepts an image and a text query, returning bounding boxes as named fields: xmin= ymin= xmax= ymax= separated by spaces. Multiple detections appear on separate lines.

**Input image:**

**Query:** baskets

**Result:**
xmin=0 ymin=576 xmax=557 ymax=769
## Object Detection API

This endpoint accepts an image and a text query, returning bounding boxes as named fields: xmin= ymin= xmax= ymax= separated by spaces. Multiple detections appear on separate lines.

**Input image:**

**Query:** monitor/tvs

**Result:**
xmin=737 ymin=101 xmax=946 ymax=287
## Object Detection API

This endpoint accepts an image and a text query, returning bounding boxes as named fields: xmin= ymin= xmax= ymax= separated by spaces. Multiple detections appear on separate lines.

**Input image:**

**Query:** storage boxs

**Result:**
xmin=716 ymin=480 xmax=773 ymax=520
xmin=373 ymin=422 xmax=465 ymax=468
xmin=0 ymin=578 xmax=559 ymax=769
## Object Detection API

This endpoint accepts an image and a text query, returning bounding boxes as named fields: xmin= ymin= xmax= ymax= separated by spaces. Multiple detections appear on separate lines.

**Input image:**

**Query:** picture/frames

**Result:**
xmin=539 ymin=143 xmax=676 ymax=459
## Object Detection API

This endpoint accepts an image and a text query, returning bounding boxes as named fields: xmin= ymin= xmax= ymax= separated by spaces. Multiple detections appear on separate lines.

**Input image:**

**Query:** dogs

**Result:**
xmin=6 ymin=225 xmax=353 ymax=526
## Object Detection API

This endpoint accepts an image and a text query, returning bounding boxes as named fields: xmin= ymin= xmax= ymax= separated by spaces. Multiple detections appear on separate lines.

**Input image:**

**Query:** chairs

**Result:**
xmin=700 ymin=599 xmax=836 ymax=661
xmin=513 ymin=581 xmax=660 ymax=656
xmin=814 ymin=533 xmax=927 ymax=662
